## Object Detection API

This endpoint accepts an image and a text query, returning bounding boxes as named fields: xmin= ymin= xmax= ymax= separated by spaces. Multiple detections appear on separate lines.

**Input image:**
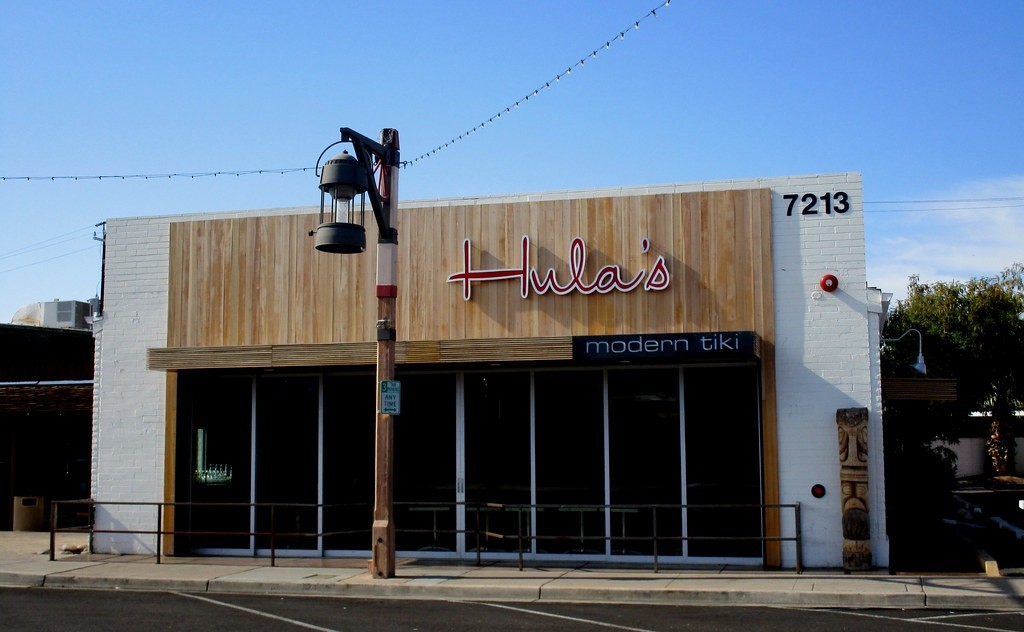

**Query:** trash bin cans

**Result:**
xmin=13 ymin=496 xmax=44 ymax=532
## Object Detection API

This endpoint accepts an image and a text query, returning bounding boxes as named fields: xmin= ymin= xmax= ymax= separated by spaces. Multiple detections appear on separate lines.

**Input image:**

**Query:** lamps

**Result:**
xmin=880 ymin=328 xmax=927 ymax=375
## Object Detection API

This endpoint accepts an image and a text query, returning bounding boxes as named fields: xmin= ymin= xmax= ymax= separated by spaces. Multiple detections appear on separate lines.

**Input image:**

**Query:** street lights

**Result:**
xmin=306 ymin=127 xmax=401 ymax=580
